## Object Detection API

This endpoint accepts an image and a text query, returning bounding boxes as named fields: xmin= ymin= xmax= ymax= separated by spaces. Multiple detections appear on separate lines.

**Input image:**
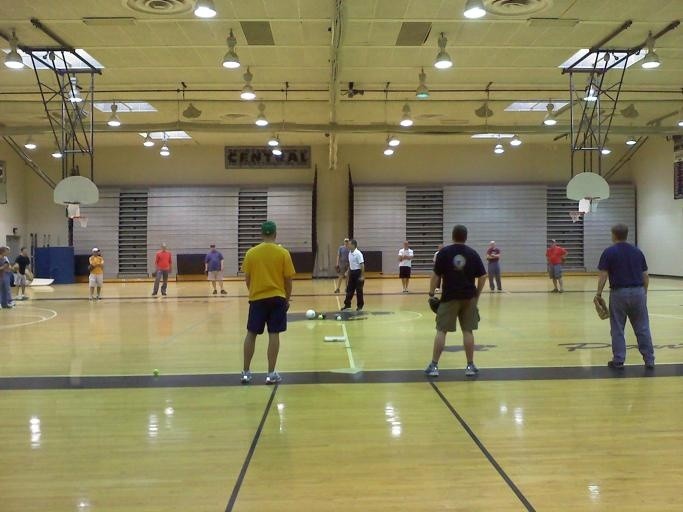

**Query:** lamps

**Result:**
xmin=185 ymin=0 xmax=282 ymax=157
xmin=142 ymin=130 xmax=154 ymax=147
xmin=159 ymin=130 xmax=170 ymax=156
xmin=108 ymin=102 xmax=120 ymax=127
xmin=4 ymin=31 xmax=24 ymax=69
xmin=24 ymin=134 xmax=36 ymax=150
xmin=67 ymin=77 xmax=82 ymax=101
xmin=51 ymin=136 xmax=63 ymax=158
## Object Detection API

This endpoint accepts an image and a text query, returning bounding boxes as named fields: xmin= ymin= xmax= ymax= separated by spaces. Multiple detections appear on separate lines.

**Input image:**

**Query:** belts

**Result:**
xmin=608 ymin=284 xmax=644 ymax=289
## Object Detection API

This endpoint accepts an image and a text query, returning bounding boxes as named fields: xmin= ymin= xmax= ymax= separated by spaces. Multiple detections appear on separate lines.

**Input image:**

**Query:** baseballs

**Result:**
xmin=323 ymin=335 xmax=347 ymax=342
xmin=153 ymin=369 xmax=159 ymax=375
xmin=318 ymin=315 xmax=323 ymax=320
xmin=337 ymin=316 xmax=341 ymax=320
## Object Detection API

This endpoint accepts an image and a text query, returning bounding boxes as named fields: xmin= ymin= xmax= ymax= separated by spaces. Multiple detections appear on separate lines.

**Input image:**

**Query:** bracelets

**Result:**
xmin=595 ymin=294 xmax=600 ymax=297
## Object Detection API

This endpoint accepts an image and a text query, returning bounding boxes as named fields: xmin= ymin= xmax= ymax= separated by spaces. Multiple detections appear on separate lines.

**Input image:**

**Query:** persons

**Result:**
xmin=333 ymin=238 xmax=349 ymax=293
xmin=431 ymin=244 xmax=443 ymax=291
xmin=0 ymin=246 xmax=15 ymax=309
xmin=423 ymin=224 xmax=486 ymax=376
xmin=485 ymin=240 xmax=502 ymax=290
xmin=12 ymin=246 xmax=32 ymax=300
xmin=240 ymin=221 xmax=295 ymax=385
xmin=592 ymin=222 xmax=656 ymax=370
xmin=151 ymin=242 xmax=172 ymax=296
xmin=340 ymin=239 xmax=364 ymax=311
xmin=544 ymin=239 xmax=567 ymax=293
xmin=87 ymin=248 xmax=104 ymax=301
xmin=397 ymin=242 xmax=413 ymax=292
xmin=204 ymin=242 xmax=227 ymax=294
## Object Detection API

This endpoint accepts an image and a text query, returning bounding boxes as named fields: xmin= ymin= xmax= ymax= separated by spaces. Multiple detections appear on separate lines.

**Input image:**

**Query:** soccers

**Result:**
xmin=305 ymin=310 xmax=315 ymax=319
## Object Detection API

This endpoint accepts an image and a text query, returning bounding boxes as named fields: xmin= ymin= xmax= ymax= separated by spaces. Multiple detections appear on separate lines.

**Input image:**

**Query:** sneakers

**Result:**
xmin=340 ymin=304 xmax=351 ymax=311
xmin=150 ymin=292 xmax=157 ymax=296
xmin=402 ymin=288 xmax=408 ymax=292
xmin=15 ymin=295 xmax=29 ymax=300
xmin=422 ymin=364 xmax=439 ymax=376
xmin=333 ymin=287 xmax=340 ymax=293
xmin=212 ymin=290 xmax=217 ymax=294
xmin=88 ymin=295 xmax=101 ymax=300
xmin=550 ymin=288 xmax=563 ymax=293
xmin=240 ymin=369 xmax=251 ymax=382
xmin=161 ymin=291 xmax=167 ymax=296
xmin=355 ymin=306 xmax=363 ymax=311
xmin=264 ymin=370 xmax=282 ymax=383
xmin=220 ymin=289 xmax=227 ymax=294
xmin=465 ymin=362 xmax=480 ymax=376
xmin=645 ymin=365 xmax=654 ymax=369
xmin=606 ymin=361 xmax=624 ymax=369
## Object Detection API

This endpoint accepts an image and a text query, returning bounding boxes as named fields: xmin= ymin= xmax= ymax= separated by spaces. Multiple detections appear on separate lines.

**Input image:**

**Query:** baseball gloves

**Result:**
xmin=88 ymin=264 xmax=93 ymax=269
xmin=429 ymin=297 xmax=440 ymax=313
xmin=334 ymin=265 xmax=339 ymax=272
xmin=593 ymin=296 xmax=609 ymax=319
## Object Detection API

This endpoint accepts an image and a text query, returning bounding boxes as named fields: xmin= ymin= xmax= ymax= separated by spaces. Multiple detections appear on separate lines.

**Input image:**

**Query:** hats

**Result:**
xmin=260 ymin=220 xmax=276 ymax=236
xmin=343 ymin=238 xmax=349 ymax=242
xmin=91 ymin=247 xmax=99 ymax=252
